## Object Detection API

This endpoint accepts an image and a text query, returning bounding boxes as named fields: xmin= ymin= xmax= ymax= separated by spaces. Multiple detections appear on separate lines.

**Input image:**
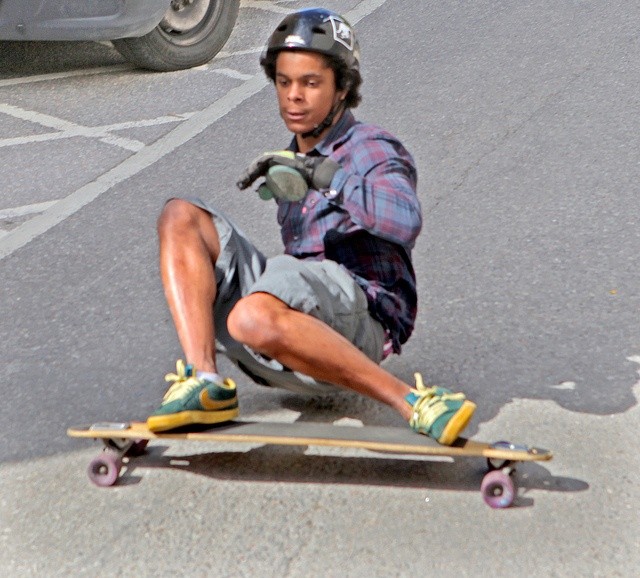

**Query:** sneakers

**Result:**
xmin=147 ymin=359 xmax=240 ymax=433
xmin=405 ymin=372 xmax=477 ymax=445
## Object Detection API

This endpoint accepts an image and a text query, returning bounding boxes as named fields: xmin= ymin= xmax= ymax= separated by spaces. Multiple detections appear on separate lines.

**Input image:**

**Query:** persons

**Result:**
xmin=145 ymin=6 xmax=478 ymax=448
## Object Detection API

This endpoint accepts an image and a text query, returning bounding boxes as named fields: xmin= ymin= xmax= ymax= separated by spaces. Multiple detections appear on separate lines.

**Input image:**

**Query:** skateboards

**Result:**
xmin=66 ymin=420 xmax=554 ymax=510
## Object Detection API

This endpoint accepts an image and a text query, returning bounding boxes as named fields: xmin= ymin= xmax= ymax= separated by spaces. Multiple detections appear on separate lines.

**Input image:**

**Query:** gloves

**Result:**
xmin=236 ymin=150 xmax=341 ymax=202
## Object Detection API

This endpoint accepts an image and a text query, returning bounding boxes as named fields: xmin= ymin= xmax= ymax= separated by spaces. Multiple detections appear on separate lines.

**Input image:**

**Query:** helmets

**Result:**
xmin=260 ymin=7 xmax=360 ymax=91
